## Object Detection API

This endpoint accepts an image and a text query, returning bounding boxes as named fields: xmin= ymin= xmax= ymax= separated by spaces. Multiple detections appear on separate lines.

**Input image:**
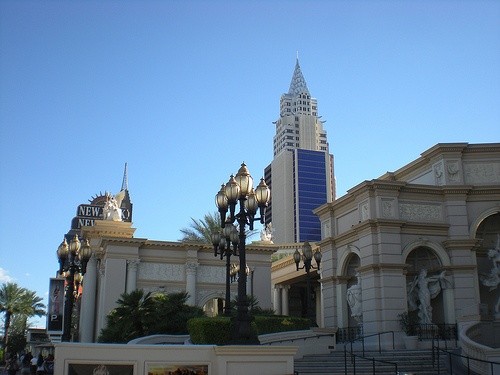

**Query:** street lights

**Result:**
xmin=56 ymin=233 xmax=93 ymax=343
xmin=210 ymin=161 xmax=271 ymax=346
xmin=293 ymin=239 xmax=322 ymax=321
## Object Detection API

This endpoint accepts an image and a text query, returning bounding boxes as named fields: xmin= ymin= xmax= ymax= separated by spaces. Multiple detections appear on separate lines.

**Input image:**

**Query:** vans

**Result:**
xmin=126 ymin=334 xmax=192 ymax=346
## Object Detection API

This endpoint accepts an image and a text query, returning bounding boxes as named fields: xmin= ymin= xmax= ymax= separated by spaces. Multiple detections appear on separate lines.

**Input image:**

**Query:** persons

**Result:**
xmin=8 ymin=352 xmax=54 ymax=375
xmin=409 ymin=270 xmax=445 ymax=324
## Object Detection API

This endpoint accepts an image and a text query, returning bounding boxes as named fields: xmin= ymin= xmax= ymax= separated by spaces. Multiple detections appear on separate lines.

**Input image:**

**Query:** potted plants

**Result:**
xmin=397 ymin=311 xmax=423 ymax=350
